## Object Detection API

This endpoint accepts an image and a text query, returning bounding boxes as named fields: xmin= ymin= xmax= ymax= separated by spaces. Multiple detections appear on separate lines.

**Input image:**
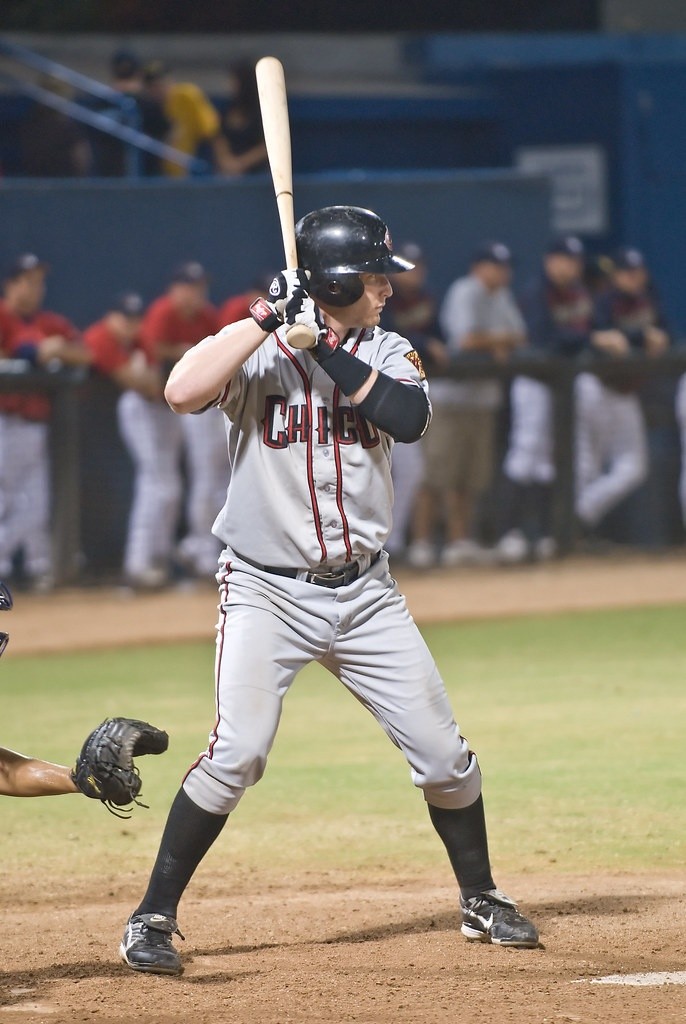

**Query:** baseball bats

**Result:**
xmin=254 ymin=54 xmax=318 ymax=351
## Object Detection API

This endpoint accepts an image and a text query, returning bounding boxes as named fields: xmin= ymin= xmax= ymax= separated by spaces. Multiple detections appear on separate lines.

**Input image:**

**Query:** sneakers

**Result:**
xmin=118 ymin=910 xmax=186 ymax=974
xmin=459 ymin=888 xmax=540 ymax=945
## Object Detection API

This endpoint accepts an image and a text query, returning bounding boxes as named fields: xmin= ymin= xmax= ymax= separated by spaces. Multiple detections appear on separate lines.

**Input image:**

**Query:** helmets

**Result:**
xmin=295 ymin=205 xmax=418 ymax=306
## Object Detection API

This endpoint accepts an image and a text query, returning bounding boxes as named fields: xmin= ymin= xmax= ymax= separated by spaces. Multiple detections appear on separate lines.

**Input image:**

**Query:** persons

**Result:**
xmin=0 ymin=254 xmax=88 ymax=592
xmin=119 ymin=206 xmax=540 ymax=975
xmin=54 ymin=258 xmax=292 ymax=593
xmin=376 ymin=238 xmax=670 ymax=569
xmin=0 ymin=584 xmax=169 ymax=819
xmin=81 ymin=52 xmax=271 ymax=179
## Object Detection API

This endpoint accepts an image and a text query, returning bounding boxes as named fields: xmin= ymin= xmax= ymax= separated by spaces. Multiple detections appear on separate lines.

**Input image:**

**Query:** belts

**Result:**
xmin=232 ymin=547 xmax=382 ymax=588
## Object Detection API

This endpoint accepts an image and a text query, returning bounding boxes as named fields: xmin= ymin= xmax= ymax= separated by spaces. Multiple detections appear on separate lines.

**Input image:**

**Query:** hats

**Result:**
xmin=471 ymin=242 xmax=512 ymax=261
xmin=543 ymin=236 xmax=585 ymax=256
xmin=169 ymin=261 xmax=204 ymax=284
xmin=599 ymin=246 xmax=642 ymax=270
xmin=113 ymin=293 xmax=145 ymax=315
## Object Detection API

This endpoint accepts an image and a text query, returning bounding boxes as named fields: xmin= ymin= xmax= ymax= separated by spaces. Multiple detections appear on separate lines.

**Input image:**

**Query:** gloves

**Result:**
xmin=276 ymin=289 xmax=342 ymax=362
xmin=250 ymin=268 xmax=312 ymax=334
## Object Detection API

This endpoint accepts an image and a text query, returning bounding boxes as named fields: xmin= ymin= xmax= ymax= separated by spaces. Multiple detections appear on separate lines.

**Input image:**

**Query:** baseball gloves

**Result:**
xmin=70 ymin=716 xmax=171 ymax=806
